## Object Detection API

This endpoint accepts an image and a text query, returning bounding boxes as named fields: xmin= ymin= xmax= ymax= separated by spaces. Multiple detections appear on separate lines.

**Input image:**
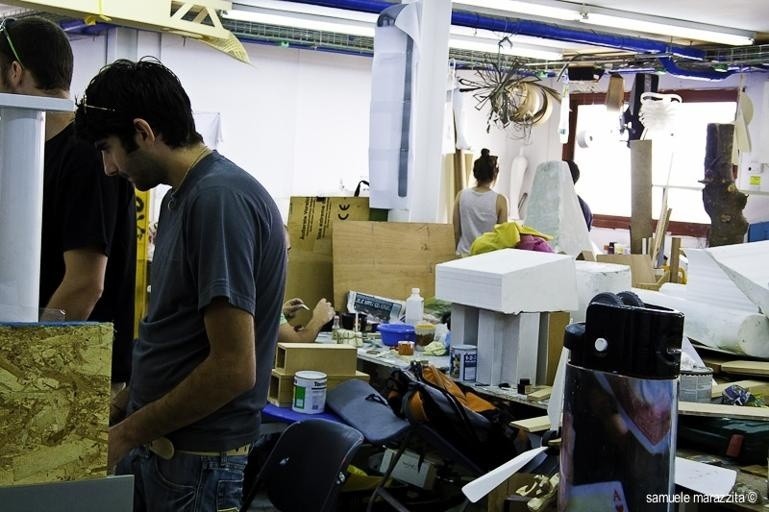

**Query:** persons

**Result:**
xmin=0 ymin=16 xmax=137 ymax=418
xmin=279 ymin=225 xmax=336 ymax=343
xmin=76 ymin=56 xmax=288 ymax=512
xmin=451 ymin=148 xmax=507 ymax=257
xmin=565 ymin=159 xmax=594 ymax=230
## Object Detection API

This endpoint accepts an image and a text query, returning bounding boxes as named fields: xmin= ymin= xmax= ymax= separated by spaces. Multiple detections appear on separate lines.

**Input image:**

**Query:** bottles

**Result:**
xmin=405 ymin=287 xmax=425 ymax=328
xmin=416 ymin=325 xmax=435 ymax=346
xmin=331 ymin=315 xmax=341 ymax=341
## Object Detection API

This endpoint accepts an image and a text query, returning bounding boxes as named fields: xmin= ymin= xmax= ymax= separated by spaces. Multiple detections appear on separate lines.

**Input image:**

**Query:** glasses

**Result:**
xmin=0 ymin=16 xmax=28 ymax=69
xmin=79 ymin=92 xmax=122 ymax=116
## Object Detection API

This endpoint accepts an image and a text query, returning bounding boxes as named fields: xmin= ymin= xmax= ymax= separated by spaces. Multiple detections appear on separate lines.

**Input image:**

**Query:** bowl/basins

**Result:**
xmin=375 ymin=322 xmax=418 ymax=349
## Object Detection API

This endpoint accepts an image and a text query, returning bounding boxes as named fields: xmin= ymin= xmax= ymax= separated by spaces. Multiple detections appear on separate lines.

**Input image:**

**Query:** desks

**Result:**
xmin=312 ymin=327 xmax=567 ymax=464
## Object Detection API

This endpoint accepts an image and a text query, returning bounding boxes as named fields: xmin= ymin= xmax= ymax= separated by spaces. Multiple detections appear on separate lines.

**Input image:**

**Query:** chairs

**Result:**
xmin=239 ymin=416 xmax=365 ymax=512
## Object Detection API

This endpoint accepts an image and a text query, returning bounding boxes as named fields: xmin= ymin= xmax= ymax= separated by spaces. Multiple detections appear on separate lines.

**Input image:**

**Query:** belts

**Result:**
xmin=131 ymin=422 xmax=252 ymax=459
